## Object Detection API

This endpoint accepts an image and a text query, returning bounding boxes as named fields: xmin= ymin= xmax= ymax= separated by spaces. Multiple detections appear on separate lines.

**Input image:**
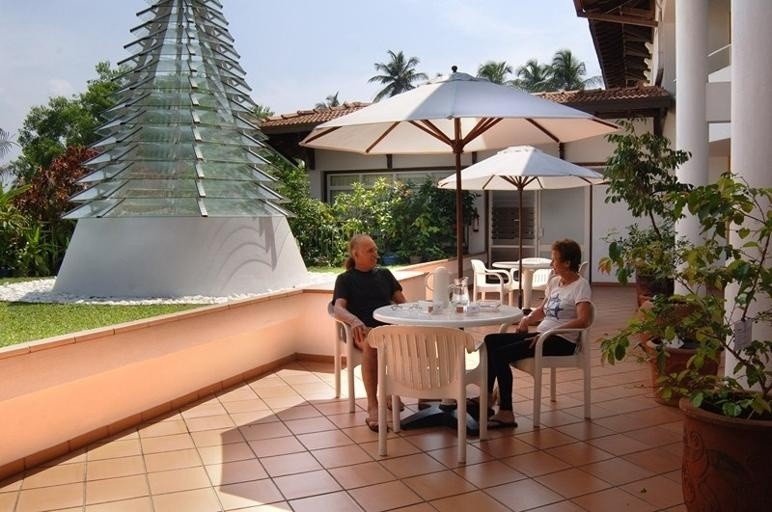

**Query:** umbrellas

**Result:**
xmin=434 ymin=144 xmax=616 ymax=266
xmin=297 ymin=66 xmax=625 ymax=295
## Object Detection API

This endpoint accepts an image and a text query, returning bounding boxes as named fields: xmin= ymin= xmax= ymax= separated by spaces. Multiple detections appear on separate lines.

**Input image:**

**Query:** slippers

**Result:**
xmin=366 ymin=418 xmax=389 ymax=432
xmin=387 ymin=399 xmax=404 ymax=411
xmin=487 ymin=418 xmax=517 ymax=429
xmin=466 ymin=398 xmax=495 ymax=416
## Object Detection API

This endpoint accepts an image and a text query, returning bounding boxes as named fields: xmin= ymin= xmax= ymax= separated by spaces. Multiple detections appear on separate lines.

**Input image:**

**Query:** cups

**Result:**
xmin=455 ymin=305 xmax=464 ymax=313
xmin=427 ymin=305 xmax=432 ymax=313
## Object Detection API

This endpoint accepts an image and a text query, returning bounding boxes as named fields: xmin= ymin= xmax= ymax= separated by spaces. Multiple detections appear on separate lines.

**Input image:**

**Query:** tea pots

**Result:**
xmin=424 ymin=266 xmax=452 ymax=308
xmin=447 ymin=277 xmax=470 ymax=306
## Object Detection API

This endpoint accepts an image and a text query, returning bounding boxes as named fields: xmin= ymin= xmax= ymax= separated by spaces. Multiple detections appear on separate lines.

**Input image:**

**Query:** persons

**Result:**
xmin=331 ymin=231 xmax=409 ymax=432
xmin=471 ymin=238 xmax=595 ymax=427
